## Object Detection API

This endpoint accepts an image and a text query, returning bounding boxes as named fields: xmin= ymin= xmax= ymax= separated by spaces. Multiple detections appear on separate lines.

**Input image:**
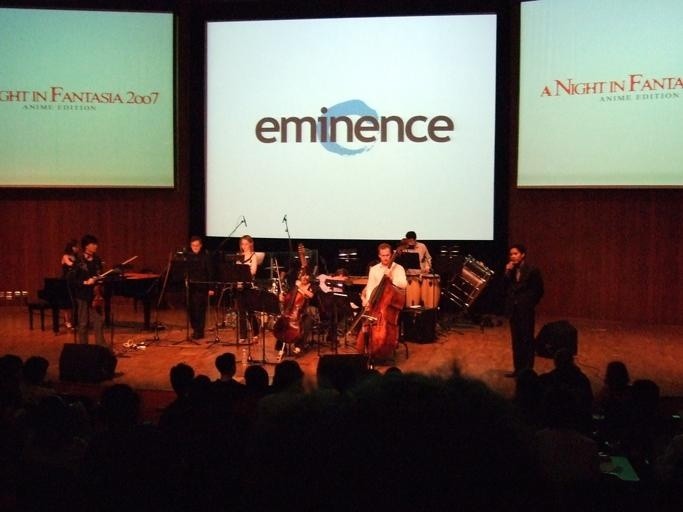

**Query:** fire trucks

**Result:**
xmin=316 ymin=276 xmax=349 ymax=281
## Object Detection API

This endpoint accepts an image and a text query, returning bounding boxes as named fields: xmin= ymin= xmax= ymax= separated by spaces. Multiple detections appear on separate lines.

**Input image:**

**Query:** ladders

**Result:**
xmin=91 ymin=257 xmax=105 ymax=310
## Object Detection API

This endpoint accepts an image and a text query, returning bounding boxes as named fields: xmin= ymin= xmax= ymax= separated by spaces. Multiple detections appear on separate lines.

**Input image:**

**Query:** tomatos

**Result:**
xmin=37 ymin=273 xmax=162 ymax=336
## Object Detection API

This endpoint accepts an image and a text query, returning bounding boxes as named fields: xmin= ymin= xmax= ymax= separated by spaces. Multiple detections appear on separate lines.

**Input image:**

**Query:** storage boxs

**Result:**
xmin=28 ymin=300 xmax=79 ymax=331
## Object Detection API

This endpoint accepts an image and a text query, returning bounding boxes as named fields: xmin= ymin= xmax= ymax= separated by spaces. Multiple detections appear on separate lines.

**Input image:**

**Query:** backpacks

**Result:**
xmin=421 ymin=274 xmax=440 ymax=310
xmin=267 ymin=278 xmax=286 ymax=295
xmin=406 ymin=274 xmax=421 ymax=308
xmin=442 ymin=254 xmax=495 ymax=311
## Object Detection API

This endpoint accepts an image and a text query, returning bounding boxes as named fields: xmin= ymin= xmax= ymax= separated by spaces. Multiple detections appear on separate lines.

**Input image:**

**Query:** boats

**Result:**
xmin=59 ymin=343 xmax=118 ymax=384
xmin=402 ymin=308 xmax=436 ymax=344
xmin=316 ymin=354 xmax=367 ymax=391
xmin=535 ymin=319 xmax=578 ymax=359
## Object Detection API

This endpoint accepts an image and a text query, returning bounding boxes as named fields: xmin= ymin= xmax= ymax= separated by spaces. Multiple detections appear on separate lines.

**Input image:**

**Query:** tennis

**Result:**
xmin=273 ymin=261 xmax=318 ymax=343
xmin=355 ymin=246 xmax=406 ymax=362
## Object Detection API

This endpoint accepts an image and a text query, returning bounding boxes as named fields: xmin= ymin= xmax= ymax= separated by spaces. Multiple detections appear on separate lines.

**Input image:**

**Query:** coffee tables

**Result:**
xmin=265 ymin=266 xmax=284 ymax=269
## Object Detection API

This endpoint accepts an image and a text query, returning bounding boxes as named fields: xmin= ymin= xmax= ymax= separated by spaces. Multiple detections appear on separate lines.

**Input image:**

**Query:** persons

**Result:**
xmin=61 ymin=239 xmax=82 ymax=332
xmin=70 ymin=234 xmax=124 ymax=347
xmin=396 ymin=230 xmax=434 ymax=282
xmin=235 ymin=234 xmax=260 ymax=345
xmin=274 ymin=267 xmax=320 ymax=358
xmin=0 ymin=318 xmax=682 ymax=512
xmin=503 ymin=243 xmax=545 ymax=377
xmin=360 ymin=242 xmax=409 ymax=350
xmin=184 ymin=236 xmax=216 ymax=339
xmin=315 ymin=268 xmax=360 ymax=346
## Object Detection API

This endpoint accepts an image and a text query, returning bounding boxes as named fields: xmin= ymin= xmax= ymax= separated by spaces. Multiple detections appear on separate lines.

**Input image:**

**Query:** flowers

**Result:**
xmin=282 ymin=214 xmax=287 ymax=223
xmin=243 ymin=216 xmax=247 ymax=227
xmin=421 ymin=251 xmax=427 ymax=262
